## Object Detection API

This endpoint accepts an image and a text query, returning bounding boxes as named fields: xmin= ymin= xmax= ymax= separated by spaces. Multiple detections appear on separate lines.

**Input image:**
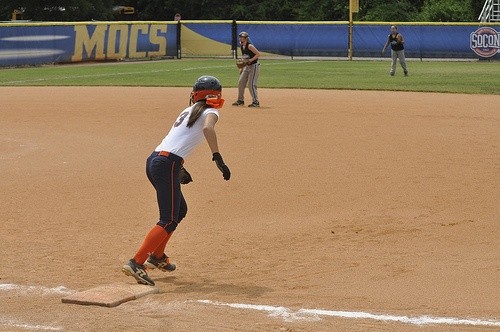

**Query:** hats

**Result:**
xmin=239 ymin=32 xmax=248 ymax=37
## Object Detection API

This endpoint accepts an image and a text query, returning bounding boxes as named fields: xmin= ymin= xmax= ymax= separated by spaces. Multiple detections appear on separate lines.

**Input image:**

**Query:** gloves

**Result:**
xmin=212 ymin=152 xmax=231 ymax=181
xmin=179 ymin=166 xmax=193 ymax=184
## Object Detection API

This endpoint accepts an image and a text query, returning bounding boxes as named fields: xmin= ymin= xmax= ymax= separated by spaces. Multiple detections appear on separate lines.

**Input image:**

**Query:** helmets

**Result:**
xmin=191 ymin=76 xmax=222 ymax=104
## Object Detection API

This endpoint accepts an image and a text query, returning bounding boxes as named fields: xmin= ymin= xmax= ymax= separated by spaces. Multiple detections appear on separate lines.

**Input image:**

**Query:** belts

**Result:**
xmin=157 ymin=151 xmax=184 ymax=165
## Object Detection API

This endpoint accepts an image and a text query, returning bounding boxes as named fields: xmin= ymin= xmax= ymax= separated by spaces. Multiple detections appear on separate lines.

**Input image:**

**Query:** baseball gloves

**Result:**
xmin=235 ymin=58 xmax=249 ymax=67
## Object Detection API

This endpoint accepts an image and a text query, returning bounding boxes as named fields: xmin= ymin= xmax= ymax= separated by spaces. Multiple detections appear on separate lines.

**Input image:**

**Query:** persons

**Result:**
xmin=121 ymin=76 xmax=231 ymax=286
xmin=382 ymin=25 xmax=408 ymax=77
xmin=231 ymin=32 xmax=261 ymax=107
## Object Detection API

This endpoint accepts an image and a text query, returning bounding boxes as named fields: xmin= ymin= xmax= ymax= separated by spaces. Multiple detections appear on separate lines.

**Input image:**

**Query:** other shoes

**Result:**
xmin=248 ymin=101 xmax=260 ymax=107
xmin=232 ymin=100 xmax=244 ymax=105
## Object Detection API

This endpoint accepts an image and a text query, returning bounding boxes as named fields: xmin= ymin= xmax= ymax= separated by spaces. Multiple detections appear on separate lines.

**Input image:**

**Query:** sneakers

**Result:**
xmin=145 ymin=253 xmax=176 ymax=272
xmin=122 ymin=259 xmax=155 ymax=286
